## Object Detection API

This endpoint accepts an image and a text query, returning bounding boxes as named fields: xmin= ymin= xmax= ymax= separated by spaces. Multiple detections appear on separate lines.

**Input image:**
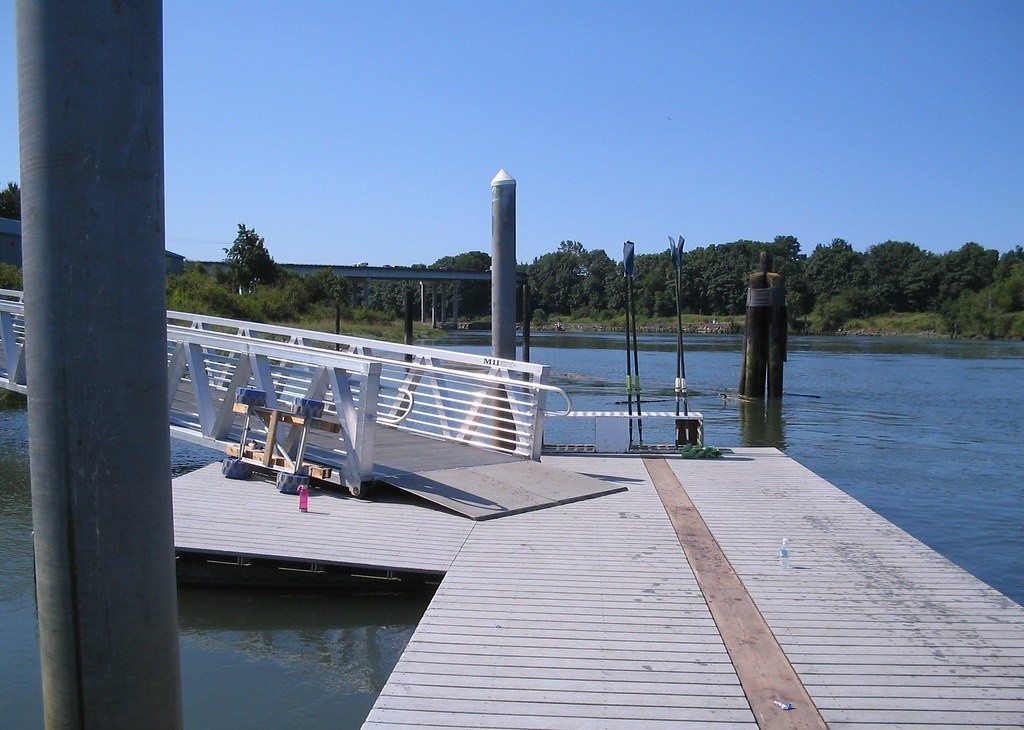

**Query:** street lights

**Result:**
xmin=419 ymin=281 xmax=423 ymax=322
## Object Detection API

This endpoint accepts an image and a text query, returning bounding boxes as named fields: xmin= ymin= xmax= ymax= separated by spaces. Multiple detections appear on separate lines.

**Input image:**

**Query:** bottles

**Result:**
xmin=297 ymin=485 xmax=309 ymax=512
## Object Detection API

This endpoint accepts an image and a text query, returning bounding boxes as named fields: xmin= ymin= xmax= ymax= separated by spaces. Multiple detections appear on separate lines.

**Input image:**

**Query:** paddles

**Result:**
xmin=668 ymin=235 xmax=688 ymax=416
xmin=624 ymin=241 xmax=644 ymax=449
